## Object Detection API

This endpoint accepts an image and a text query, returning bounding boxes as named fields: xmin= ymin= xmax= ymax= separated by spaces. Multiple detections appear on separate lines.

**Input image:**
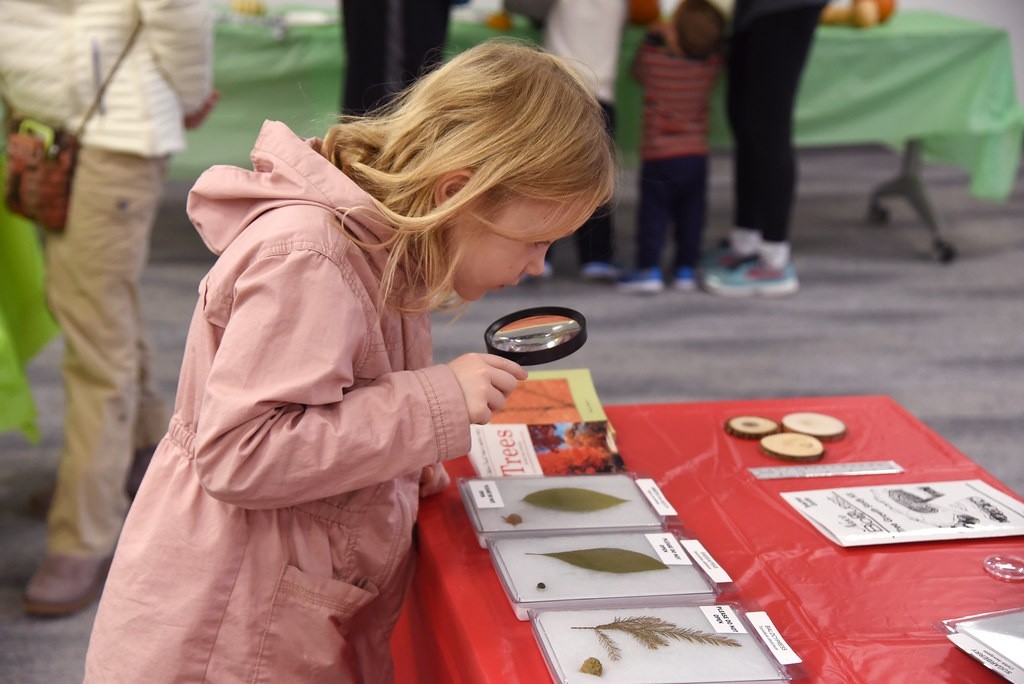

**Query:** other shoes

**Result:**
xmin=525 ymin=264 xmax=552 ymax=282
xmin=583 ymin=260 xmax=616 ymax=278
xmin=23 ymin=557 xmax=110 ymax=615
xmin=27 ymin=446 xmax=156 ymax=521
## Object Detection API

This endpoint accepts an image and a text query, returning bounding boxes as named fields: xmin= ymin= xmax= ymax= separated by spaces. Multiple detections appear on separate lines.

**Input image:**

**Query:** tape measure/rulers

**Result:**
xmin=746 ymin=460 xmax=905 ymax=479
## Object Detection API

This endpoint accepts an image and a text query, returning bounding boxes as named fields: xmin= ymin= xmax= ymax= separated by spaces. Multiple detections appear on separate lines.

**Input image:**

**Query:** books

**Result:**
xmin=465 ymin=369 xmax=628 ymax=480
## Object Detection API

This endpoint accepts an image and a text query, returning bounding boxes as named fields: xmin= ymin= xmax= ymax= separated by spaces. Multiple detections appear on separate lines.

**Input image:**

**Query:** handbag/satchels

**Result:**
xmin=5 ymin=117 xmax=80 ymax=234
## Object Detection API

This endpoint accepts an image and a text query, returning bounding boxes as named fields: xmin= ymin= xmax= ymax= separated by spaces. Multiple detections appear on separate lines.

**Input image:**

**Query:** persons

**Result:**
xmin=504 ymin=0 xmax=832 ymax=300
xmin=340 ymin=0 xmax=470 ymax=124
xmin=82 ymin=37 xmax=617 ymax=684
xmin=0 ymin=0 xmax=219 ymax=621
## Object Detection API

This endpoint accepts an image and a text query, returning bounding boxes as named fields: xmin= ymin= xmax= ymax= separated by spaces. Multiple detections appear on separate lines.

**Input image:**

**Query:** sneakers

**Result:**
xmin=665 ymin=263 xmax=698 ymax=289
xmin=703 ymin=254 xmax=800 ymax=296
xmin=619 ymin=263 xmax=661 ymax=289
xmin=703 ymin=238 xmax=751 ymax=269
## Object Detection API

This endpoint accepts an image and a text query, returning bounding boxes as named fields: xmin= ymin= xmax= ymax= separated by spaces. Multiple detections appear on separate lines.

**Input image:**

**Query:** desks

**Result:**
xmin=384 ymin=394 xmax=1024 ymax=684
xmin=171 ymin=0 xmax=1024 ymax=268
